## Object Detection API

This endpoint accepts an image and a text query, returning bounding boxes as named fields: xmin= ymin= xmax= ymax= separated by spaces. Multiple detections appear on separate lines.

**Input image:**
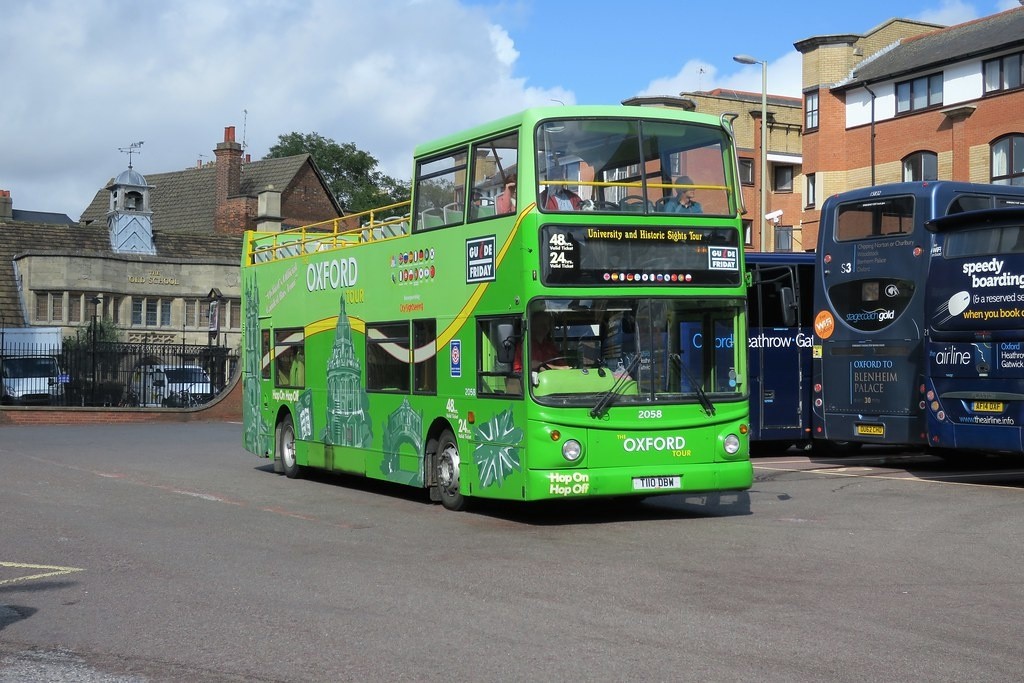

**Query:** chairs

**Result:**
xmin=252 ymin=194 xmax=675 ymax=262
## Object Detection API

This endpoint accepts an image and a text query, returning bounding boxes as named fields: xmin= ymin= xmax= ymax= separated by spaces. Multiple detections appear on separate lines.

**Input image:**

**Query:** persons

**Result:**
xmin=540 ymin=168 xmax=584 ymax=211
xmin=495 ymin=173 xmax=517 ymax=215
xmin=664 ymin=176 xmax=703 ymax=214
xmin=514 ymin=314 xmax=573 ymax=393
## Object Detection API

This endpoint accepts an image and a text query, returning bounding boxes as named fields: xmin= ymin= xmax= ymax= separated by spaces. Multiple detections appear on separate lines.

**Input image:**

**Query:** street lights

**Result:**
xmin=731 ymin=54 xmax=769 ymax=253
xmin=89 ymin=296 xmax=101 ymax=407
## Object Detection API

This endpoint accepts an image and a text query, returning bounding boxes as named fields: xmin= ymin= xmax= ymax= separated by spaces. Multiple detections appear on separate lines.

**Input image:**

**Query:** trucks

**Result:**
xmin=0 ymin=325 xmax=66 ymax=408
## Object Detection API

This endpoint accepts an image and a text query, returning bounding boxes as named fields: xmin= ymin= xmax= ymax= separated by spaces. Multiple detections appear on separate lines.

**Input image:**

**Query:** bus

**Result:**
xmin=661 ymin=247 xmax=816 ymax=455
xmin=922 ymin=204 xmax=1024 ymax=459
xmin=811 ymin=178 xmax=1024 ymax=448
xmin=238 ymin=98 xmax=800 ymax=513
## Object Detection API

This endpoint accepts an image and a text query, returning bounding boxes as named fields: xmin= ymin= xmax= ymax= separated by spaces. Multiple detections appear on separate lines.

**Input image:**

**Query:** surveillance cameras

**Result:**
xmin=765 ymin=209 xmax=783 ymax=220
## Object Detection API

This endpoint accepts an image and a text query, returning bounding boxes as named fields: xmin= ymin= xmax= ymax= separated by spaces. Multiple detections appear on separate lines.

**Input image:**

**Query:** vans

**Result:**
xmin=130 ymin=364 xmax=220 ymax=409
